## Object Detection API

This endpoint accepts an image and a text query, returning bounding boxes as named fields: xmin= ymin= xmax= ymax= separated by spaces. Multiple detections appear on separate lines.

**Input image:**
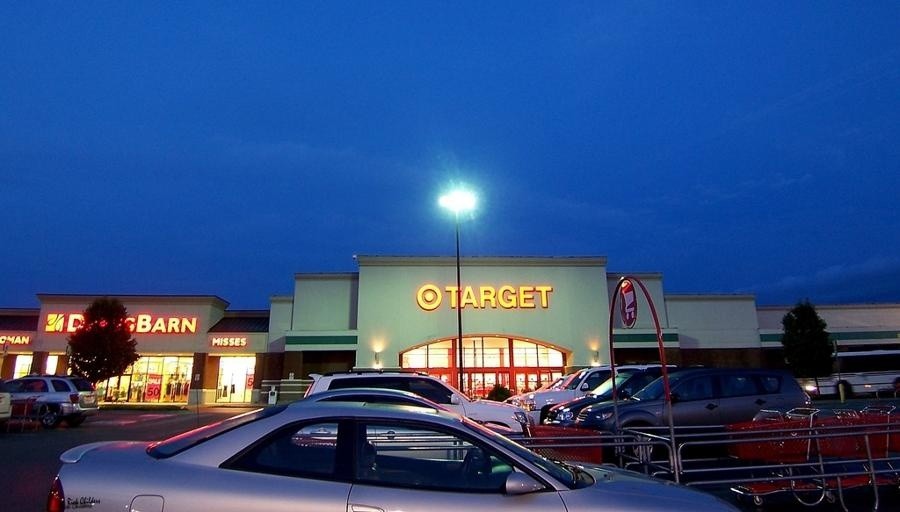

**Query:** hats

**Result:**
xmin=294 ymin=387 xmax=525 ymax=462
xmin=46 ymin=403 xmax=740 ymax=512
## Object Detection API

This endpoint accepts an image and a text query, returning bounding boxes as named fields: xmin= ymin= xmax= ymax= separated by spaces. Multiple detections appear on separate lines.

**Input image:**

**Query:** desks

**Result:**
xmin=439 ymin=190 xmax=476 ymax=392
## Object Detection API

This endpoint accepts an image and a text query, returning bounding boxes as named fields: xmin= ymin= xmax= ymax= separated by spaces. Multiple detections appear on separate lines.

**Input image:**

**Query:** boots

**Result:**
xmin=293 ymin=373 xmax=535 ymax=459
xmin=0 ymin=375 xmax=99 ymax=427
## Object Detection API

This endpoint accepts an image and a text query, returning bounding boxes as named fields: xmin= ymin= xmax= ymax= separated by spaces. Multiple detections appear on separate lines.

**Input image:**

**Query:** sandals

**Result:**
xmin=797 ymin=349 xmax=900 ymax=398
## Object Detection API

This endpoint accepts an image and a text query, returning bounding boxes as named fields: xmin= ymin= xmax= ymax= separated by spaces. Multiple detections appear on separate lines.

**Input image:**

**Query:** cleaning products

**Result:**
xmin=516 ymin=411 xmax=604 ymax=467
xmin=724 ymin=403 xmax=900 ymax=507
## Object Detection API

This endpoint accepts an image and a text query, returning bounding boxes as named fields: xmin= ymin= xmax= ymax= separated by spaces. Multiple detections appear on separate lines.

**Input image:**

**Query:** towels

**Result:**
xmin=268 ymin=391 xmax=278 ymax=406
